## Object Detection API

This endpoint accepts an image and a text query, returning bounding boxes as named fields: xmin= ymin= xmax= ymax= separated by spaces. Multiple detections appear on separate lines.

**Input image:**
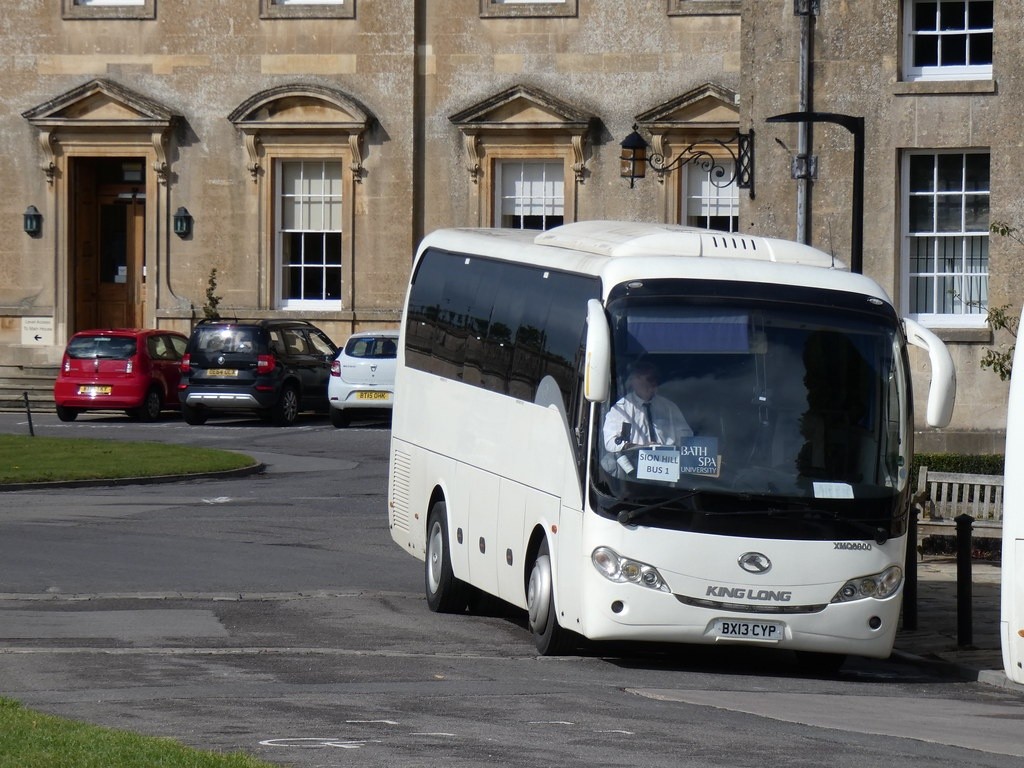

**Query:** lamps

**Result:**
xmin=23 ymin=205 xmax=42 ymax=237
xmin=620 ymin=122 xmax=756 ymax=200
xmin=173 ymin=207 xmax=192 ymax=238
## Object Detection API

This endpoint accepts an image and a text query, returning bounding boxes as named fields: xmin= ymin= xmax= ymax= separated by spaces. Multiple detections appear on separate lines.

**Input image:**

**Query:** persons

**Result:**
xmin=735 ymin=368 xmax=808 ymax=470
xmin=602 ymin=359 xmax=694 ymax=453
xmin=381 ymin=340 xmax=395 ymax=355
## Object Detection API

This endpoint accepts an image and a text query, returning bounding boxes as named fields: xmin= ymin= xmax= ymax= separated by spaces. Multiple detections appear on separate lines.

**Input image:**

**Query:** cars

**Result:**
xmin=54 ymin=328 xmax=189 ymax=423
xmin=328 ymin=329 xmax=400 ymax=429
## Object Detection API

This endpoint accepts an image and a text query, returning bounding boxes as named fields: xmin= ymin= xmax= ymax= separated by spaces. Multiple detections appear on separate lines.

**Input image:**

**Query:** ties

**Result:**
xmin=642 ymin=403 xmax=657 ymax=444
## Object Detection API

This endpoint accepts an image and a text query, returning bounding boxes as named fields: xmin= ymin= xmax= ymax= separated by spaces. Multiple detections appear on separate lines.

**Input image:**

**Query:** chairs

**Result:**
xmin=285 ymin=333 xmax=301 ymax=354
xmin=382 ymin=340 xmax=397 ymax=355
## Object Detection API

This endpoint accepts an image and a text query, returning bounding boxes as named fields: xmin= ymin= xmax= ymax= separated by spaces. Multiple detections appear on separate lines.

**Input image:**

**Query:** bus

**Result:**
xmin=388 ymin=221 xmax=957 ymax=658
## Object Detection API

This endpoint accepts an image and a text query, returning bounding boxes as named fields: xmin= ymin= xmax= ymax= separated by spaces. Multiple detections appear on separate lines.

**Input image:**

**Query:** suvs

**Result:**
xmin=178 ymin=318 xmax=343 ymax=426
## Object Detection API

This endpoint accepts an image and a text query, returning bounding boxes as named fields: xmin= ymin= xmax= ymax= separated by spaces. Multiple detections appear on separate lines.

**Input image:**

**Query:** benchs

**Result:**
xmin=910 ymin=464 xmax=1004 ymax=559
